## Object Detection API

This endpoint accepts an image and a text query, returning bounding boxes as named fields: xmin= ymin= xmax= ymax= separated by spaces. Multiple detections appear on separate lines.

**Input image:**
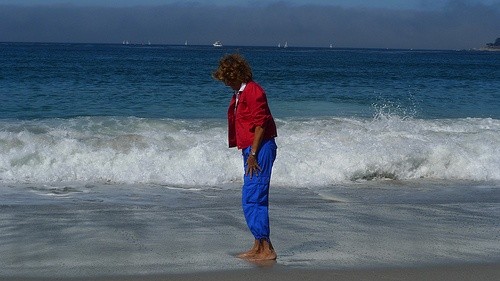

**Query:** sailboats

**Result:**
xmin=284 ymin=41 xmax=288 ymax=48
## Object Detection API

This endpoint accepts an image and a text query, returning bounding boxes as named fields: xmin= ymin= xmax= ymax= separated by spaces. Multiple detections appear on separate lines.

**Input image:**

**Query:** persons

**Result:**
xmin=212 ymin=53 xmax=279 ymax=262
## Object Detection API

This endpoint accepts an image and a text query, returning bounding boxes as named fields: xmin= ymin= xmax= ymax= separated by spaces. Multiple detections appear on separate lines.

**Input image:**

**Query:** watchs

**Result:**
xmin=250 ymin=152 xmax=255 ymax=156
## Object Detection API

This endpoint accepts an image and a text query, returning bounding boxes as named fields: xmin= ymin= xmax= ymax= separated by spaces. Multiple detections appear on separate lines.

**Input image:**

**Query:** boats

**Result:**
xmin=214 ymin=40 xmax=223 ymax=48
